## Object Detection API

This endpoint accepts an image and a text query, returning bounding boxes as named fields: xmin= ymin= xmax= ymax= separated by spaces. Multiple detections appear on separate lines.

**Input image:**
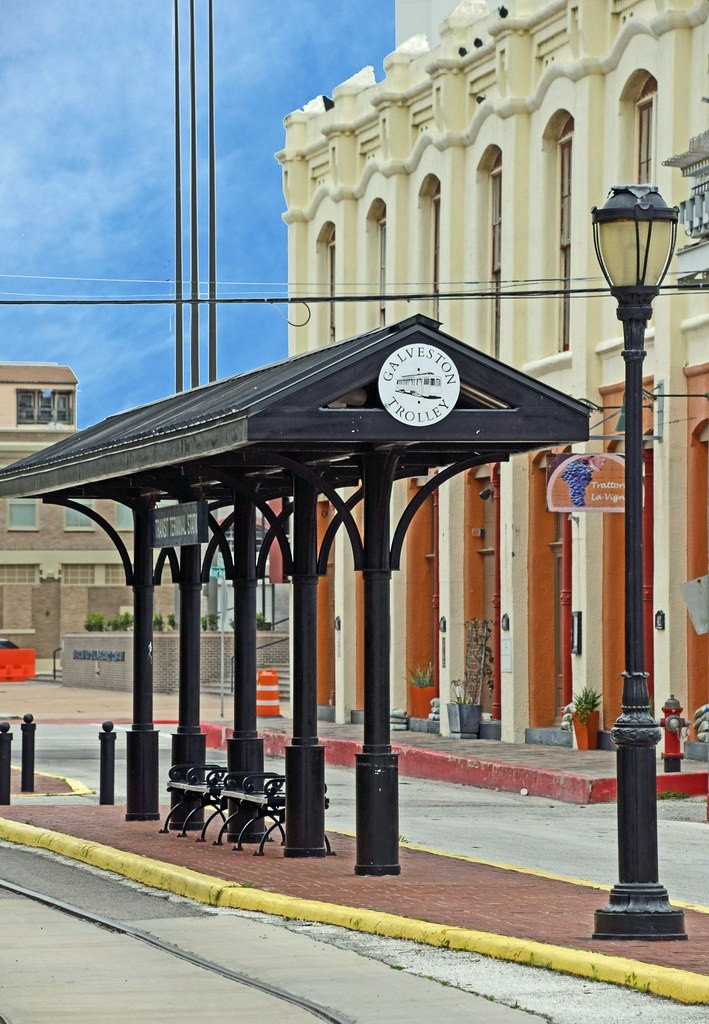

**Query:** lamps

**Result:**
xmin=478 ymin=488 xmax=493 ymax=500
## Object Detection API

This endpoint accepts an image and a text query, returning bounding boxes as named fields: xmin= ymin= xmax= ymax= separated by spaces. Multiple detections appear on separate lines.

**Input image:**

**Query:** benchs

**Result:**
xmin=158 ymin=763 xmax=275 ymax=842
xmin=212 ymin=772 xmax=338 ymax=857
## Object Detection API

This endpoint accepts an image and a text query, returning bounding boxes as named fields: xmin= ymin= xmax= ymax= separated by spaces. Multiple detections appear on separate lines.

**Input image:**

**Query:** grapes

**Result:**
xmin=561 ymin=462 xmax=594 ymax=507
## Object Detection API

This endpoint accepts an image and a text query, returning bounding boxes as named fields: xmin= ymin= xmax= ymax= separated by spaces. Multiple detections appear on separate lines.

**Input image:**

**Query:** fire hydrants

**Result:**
xmin=659 ymin=692 xmax=692 ymax=772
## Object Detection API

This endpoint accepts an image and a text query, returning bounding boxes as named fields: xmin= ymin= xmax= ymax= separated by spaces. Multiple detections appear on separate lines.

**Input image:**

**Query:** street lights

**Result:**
xmin=589 ymin=181 xmax=691 ymax=941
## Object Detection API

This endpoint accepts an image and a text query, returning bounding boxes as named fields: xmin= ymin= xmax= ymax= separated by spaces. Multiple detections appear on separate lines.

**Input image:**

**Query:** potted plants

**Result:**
xmin=446 ymin=687 xmax=489 ymax=740
xmin=400 ymin=660 xmax=435 ymax=718
xmin=570 ymin=686 xmax=604 ymax=750
